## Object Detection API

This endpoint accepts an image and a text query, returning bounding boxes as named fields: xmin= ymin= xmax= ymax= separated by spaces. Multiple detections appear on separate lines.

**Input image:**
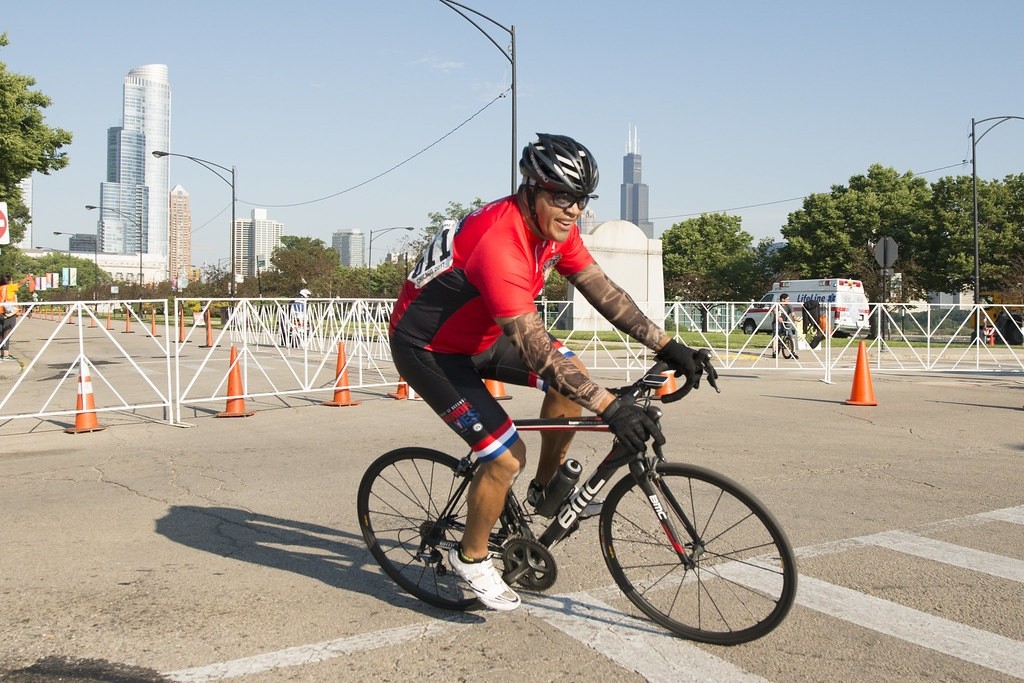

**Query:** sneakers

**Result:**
xmin=527 ymin=480 xmax=602 ymax=518
xmin=447 ymin=545 xmax=521 ymax=613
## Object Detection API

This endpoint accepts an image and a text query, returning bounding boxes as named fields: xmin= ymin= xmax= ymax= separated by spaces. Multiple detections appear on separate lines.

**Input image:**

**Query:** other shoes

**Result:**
xmin=773 ymin=353 xmax=776 ymax=357
xmin=0 ymin=354 xmax=18 ymax=362
xmin=792 ymin=355 xmax=798 ymax=359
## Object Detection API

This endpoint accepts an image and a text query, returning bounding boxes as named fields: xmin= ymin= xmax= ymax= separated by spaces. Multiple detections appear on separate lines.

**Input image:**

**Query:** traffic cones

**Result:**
xmin=64 ymin=355 xmax=105 ymax=433
xmin=387 ymin=376 xmax=421 ymax=400
xmin=173 ymin=309 xmax=192 ymax=343
xmin=198 ymin=311 xmax=221 ymax=348
xmin=214 ymin=346 xmax=255 ymax=418
xmin=484 ymin=379 xmax=513 ymax=401
xmin=33 ymin=306 xmax=163 ymax=337
xmin=321 ymin=342 xmax=362 ymax=407
xmin=846 ymin=340 xmax=879 ymax=406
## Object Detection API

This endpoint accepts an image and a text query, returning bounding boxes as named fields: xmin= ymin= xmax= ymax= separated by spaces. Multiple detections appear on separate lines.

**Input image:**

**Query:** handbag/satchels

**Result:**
xmin=4 ymin=303 xmax=18 ymax=318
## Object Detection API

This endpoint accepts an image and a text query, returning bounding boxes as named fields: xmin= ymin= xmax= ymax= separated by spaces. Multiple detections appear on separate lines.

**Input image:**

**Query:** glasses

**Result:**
xmin=539 ymin=186 xmax=589 ymax=211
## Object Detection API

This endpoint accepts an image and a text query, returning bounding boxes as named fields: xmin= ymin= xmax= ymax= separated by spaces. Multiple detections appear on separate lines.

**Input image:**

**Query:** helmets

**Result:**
xmin=519 ymin=132 xmax=599 ymax=195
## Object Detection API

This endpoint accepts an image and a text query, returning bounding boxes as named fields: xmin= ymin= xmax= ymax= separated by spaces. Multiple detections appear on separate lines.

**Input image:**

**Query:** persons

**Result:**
xmin=288 ymin=289 xmax=311 ymax=324
xmin=771 ymin=293 xmax=801 ymax=359
xmin=388 ymin=133 xmax=718 ymax=611
xmin=0 ymin=274 xmax=31 ymax=361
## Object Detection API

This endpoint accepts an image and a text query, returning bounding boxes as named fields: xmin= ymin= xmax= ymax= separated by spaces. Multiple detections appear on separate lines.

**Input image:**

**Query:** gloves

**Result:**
xmin=659 ymin=337 xmax=703 ymax=390
xmin=595 ymin=397 xmax=666 ymax=455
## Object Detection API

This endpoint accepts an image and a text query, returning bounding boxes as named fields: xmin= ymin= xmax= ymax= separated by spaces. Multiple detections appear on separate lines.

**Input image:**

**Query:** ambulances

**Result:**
xmin=740 ymin=278 xmax=871 ymax=338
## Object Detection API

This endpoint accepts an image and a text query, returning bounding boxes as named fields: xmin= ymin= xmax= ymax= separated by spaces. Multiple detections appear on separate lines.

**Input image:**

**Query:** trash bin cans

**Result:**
xmin=994 ymin=312 xmax=1022 ymax=345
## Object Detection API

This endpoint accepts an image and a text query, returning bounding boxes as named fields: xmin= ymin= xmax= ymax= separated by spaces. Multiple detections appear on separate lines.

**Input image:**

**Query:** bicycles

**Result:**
xmin=357 ymin=348 xmax=797 ymax=647
xmin=772 ymin=321 xmax=794 ymax=360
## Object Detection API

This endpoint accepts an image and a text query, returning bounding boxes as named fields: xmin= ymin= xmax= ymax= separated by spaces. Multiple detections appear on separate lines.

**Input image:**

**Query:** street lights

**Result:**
xmin=53 ymin=232 xmax=97 ymax=311
xmin=368 ymin=227 xmax=414 ymax=298
xmin=176 ymin=265 xmax=195 ymax=293
xmin=86 ymin=205 xmax=143 ymax=319
xmin=152 ymin=151 xmax=235 ymax=312
xmin=36 ymin=247 xmax=71 ymax=285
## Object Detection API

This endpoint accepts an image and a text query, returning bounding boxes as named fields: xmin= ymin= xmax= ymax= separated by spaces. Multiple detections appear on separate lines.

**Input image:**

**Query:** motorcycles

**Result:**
xmin=277 ymin=303 xmax=315 ymax=350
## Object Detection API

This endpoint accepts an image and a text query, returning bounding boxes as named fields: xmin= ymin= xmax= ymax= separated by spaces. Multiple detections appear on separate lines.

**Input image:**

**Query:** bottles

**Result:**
xmin=535 ymin=459 xmax=583 ymax=518
